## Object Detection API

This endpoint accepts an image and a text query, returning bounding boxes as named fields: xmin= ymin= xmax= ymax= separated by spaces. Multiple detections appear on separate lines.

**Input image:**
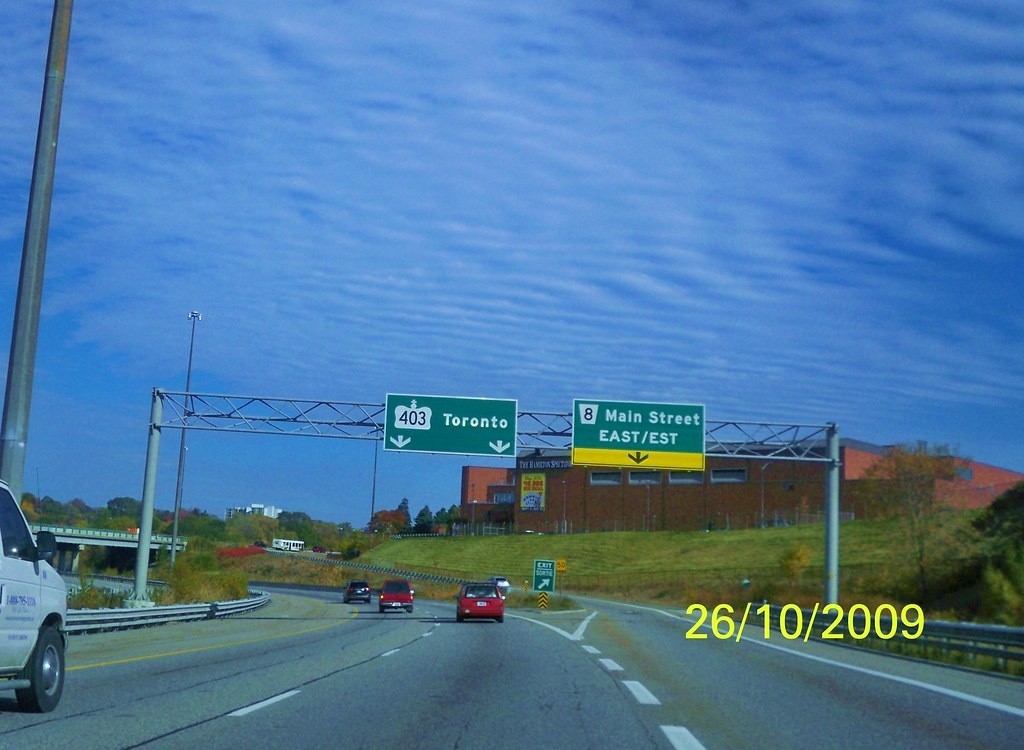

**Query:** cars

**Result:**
xmin=454 ymin=584 xmax=505 ymax=623
xmin=488 ymin=576 xmax=510 ymax=587
xmin=313 ymin=547 xmax=325 ymax=553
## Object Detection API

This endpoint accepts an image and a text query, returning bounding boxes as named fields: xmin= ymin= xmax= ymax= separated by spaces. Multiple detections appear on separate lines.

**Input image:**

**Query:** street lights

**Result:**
xmin=169 ymin=310 xmax=202 ymax=567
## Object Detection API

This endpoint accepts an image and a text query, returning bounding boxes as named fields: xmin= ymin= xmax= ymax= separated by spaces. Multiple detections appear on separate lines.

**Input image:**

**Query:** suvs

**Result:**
xmin=344 ymin=580 xmax=371 ymax=603
xmin=379 ymin=578 xmax=414 ymax=614
xmin=0 ymin=479 xmax=66 ymax=712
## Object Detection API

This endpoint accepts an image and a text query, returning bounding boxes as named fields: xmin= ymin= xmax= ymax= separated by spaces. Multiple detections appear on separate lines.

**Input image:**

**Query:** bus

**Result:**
xmin=272 ymin=538 xmax=305 ymax=552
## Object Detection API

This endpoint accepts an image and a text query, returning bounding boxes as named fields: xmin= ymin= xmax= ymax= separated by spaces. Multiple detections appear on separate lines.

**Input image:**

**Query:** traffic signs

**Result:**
xmin=533 ymin=560 xmax=556 ymax=591
xmin=381 ymin=393 xmax=519 ymax=457
xmin=570 ymin=399 xmax=708 ymax=471
